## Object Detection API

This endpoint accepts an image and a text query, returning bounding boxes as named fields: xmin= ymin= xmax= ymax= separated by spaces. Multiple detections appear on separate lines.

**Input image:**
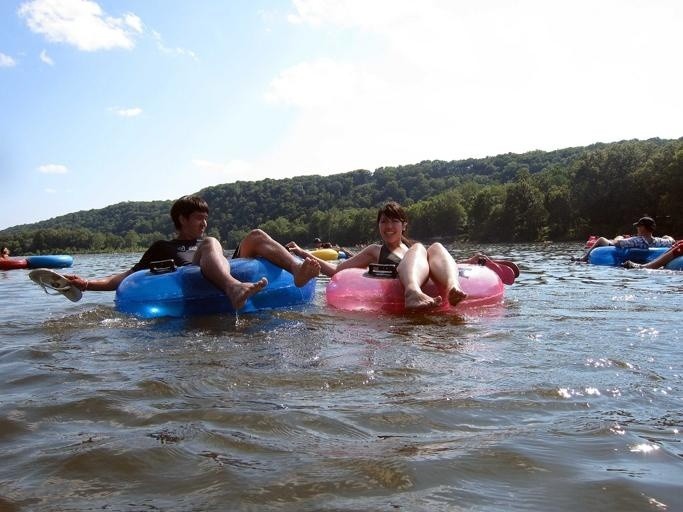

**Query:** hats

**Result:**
xmin=313 ymin=237 xmax=321 ymax=243
xmin=632 ymin=217 xmax=657 ymax=231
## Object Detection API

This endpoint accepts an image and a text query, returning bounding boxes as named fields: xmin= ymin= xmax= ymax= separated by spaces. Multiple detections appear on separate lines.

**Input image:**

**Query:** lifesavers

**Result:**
xmin=0 ymin=255 xmax=73 ymax=269
xmin=309 ymin=248 xmax=348 ymax=261
xmin=586 ymin=236 xmax=683 ymax=272
xmin=326 ymin=262 xmax=505 ymax=315
xmin=114 ymin=257 xmax=317 ymax=322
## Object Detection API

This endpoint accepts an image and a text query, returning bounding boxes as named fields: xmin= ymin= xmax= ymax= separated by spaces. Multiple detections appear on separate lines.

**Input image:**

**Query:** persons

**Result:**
xmin=0 ymin=247 xmax=12 ymax=261
xmin=60 ymin=196 xmax=321 ymax=310
xmin=570 ymin=216 xmax=683 ymax=270
xmin=286 ymin=201 xmax=488 ymax=311
xmin=310 ymin=235 xmax=353 ymax=259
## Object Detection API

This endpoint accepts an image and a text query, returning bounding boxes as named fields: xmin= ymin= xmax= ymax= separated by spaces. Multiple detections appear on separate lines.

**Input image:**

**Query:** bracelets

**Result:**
xmin=84 ymin=278 xmax=88 ymax=290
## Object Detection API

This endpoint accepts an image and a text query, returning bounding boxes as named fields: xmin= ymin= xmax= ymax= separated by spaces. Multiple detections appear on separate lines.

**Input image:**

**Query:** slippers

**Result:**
xmin=477 ymin=253 xmax=520 ymax=286
xmin=28 ymin=267 xmax=83 ymax=303
